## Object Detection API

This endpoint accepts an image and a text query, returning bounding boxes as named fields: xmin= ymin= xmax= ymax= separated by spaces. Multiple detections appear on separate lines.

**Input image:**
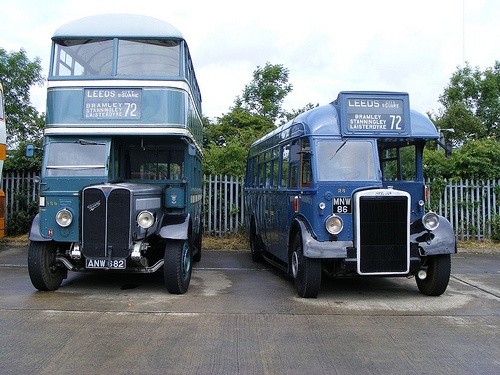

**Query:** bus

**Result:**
xmin=243 ymin=90 xmax=457 ymax=298
xmin=27 ymin=14 xmax=206 ymax=294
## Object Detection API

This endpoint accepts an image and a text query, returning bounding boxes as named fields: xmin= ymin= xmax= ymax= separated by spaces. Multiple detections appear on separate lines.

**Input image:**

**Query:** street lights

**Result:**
xmin=437 ymin=128 xmax=455 ymax=151
